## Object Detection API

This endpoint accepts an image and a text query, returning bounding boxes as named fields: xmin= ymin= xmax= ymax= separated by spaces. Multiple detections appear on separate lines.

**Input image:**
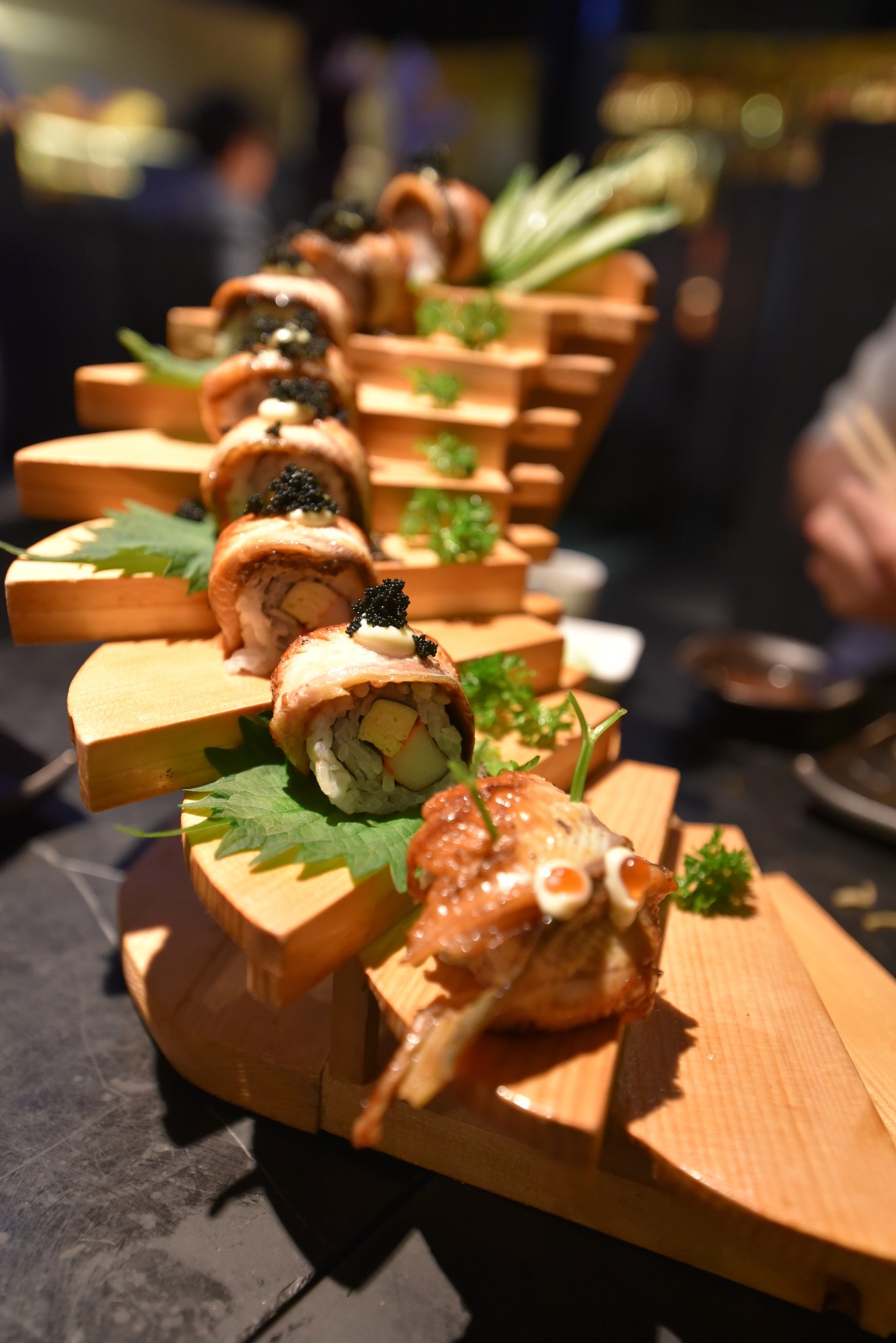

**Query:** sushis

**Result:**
xmin=197 ymin=164 xmax=679 ymax=1143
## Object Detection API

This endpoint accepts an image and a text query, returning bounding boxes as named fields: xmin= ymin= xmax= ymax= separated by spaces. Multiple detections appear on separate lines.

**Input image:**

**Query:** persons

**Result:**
xmin=788 ymin=318 xmax=896 ymax=628
xmin=136 ymin=84 xmax=278 ymax=288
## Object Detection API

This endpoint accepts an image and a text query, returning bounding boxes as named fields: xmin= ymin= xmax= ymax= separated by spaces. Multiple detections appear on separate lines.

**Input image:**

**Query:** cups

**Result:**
xmin=526 ymin=547 xmax=609 ymax=618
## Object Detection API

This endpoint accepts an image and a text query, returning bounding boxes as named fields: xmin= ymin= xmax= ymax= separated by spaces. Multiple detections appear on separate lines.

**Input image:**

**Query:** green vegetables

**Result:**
xmin=0 ymin=160 xmax=748 ymax=921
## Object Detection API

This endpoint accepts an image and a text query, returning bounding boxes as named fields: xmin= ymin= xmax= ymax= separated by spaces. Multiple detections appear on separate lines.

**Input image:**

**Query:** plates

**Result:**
xmin=681 ymin=628 xmax=862 ymax=706
xmin=555 ymin=614 xmax=646 ymax=692
